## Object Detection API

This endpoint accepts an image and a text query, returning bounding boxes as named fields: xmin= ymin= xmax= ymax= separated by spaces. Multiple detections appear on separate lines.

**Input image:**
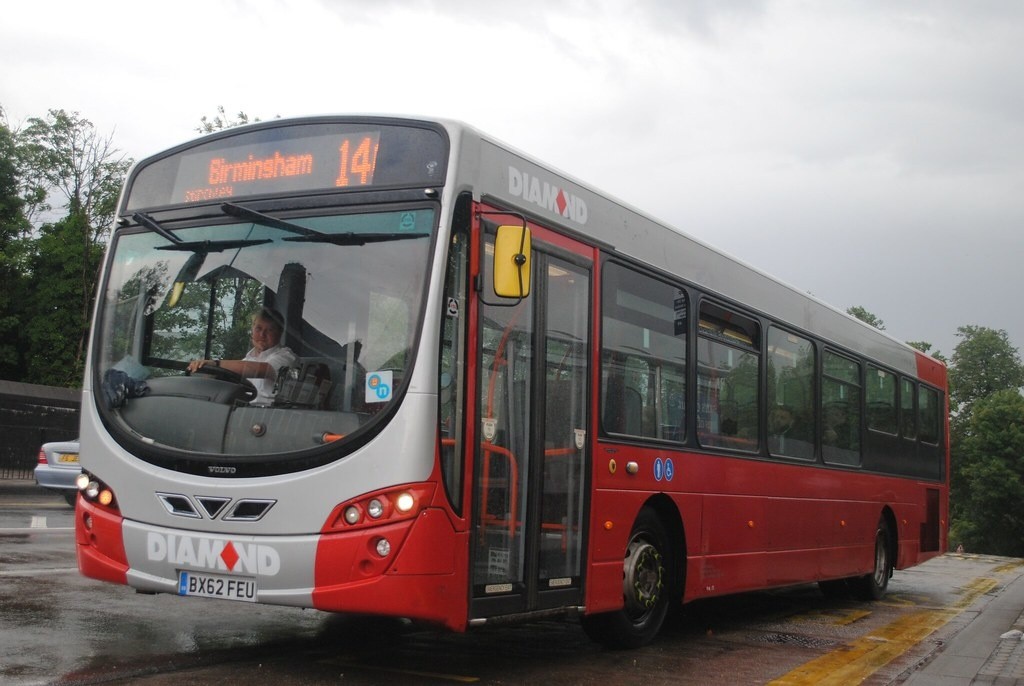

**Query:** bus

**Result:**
xmin=75 ymin=110 xmax=951 ymax=653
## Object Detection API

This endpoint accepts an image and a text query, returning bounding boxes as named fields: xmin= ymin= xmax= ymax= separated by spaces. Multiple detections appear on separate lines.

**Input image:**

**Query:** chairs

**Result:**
xmin=624 ymin=386 xmax=643 ymax=437
xmin=717 ymin=399 xmax=895 ymax=450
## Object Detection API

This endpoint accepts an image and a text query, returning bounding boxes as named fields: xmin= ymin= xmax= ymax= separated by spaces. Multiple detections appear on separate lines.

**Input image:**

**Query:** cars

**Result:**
xmin=33 ymin=436 xmax=81 ymax=506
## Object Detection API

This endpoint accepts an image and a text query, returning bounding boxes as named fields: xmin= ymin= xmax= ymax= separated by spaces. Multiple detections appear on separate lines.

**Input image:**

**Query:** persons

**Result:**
xmin=188 ymin=308 xmax=299 ymax=406
xmin=773 ymin=404 xmax=794 ymax=431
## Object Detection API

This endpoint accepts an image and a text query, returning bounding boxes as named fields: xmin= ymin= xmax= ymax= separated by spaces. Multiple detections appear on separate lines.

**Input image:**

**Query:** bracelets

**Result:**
xmin=215 ymin=360 xmax=219 ymax=366
